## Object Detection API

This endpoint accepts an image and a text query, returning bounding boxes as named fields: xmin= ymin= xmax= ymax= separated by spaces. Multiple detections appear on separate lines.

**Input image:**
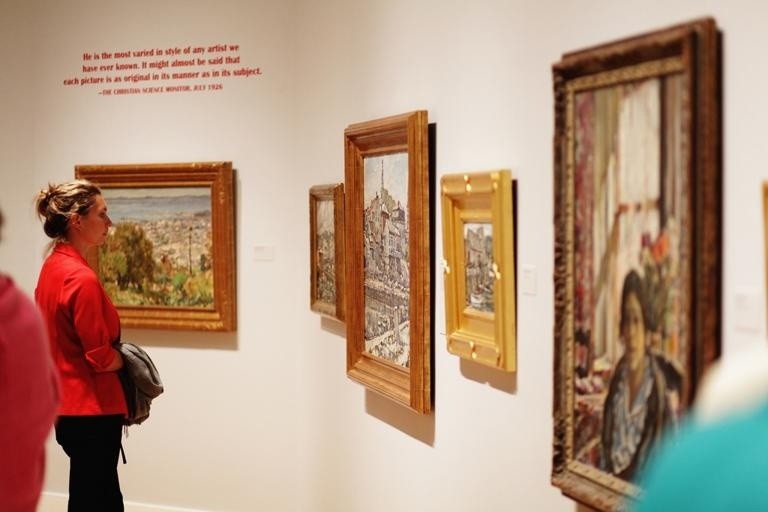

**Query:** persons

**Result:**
xmin=617 ymin=341 xmax=767 ymax=510
xmin=0 ymin=211 xmax=61 ymax=512
xmin=597 ymin=269 xmax=678 ymax=484
xmin=36 ymin=178 xmax=131 ymax=512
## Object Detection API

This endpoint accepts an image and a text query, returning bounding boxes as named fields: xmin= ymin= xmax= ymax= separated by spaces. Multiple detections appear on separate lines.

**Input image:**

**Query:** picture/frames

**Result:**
xmin=73 ymin=161 xmax=237 ymax=334
xmin=550 ymin=13 xmax=722 ymax=512
xmin=308 ymin=108 xmax=431 ymax=416
xmin=439 ymin=168 xmax=517 ymax=372
